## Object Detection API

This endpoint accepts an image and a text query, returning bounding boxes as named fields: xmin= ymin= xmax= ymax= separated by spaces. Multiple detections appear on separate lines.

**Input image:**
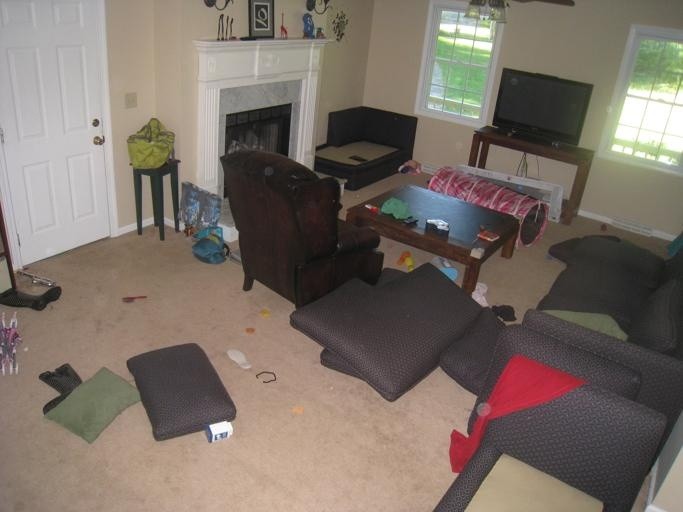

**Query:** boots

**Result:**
xmin=39 ymin=363 xmax=82 ymax=415
xmin=1 ymin=286 xmax=61 ymax=310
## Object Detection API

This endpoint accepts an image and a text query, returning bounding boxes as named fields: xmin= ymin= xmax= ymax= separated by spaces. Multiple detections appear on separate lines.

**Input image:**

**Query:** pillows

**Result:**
xmin=44 ymin=365 xmax=142 ymax=445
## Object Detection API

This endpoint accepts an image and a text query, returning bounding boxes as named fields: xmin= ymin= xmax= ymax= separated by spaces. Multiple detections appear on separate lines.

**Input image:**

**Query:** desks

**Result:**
xmin=468 ymin=125 xmax=594 ymax=225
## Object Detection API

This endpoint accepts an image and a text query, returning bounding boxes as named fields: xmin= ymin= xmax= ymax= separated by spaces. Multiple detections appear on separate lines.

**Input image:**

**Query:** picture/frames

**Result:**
xmin=247 ymin=1 xmax=274 ymax=39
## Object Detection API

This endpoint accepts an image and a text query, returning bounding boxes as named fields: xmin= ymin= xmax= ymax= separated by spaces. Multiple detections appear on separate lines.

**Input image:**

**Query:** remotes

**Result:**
xmin=403 ymin=218 xmax=419 ymax=224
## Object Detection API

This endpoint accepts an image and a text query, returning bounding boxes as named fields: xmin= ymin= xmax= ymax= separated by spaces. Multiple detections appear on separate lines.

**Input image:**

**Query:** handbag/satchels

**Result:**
xmin=178 ymin=182 xmax=222 ymax=232
xmin=127 ymin=118 xmax=174 ymax=169
xmin=193 ymin=234 xmax=230 ymax=264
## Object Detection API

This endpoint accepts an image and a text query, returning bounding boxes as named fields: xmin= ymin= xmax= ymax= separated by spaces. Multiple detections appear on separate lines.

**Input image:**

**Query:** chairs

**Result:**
xmin=220 ymin=151 xmax=384 ymax=308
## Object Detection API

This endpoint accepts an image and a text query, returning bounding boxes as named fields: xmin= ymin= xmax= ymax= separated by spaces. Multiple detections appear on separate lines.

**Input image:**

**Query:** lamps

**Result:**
xmin=462 ymin=1 xmax=509 ymax=25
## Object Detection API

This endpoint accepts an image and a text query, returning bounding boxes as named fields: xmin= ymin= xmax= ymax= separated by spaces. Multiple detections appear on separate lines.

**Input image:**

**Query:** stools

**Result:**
xmin=128 ymin=158 xmax=181 ymax=241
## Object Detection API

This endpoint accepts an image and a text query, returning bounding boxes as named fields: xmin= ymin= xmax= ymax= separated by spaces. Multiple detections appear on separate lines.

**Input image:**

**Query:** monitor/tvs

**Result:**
xmin=492 ymin=68 xmax=594 ymax=149
